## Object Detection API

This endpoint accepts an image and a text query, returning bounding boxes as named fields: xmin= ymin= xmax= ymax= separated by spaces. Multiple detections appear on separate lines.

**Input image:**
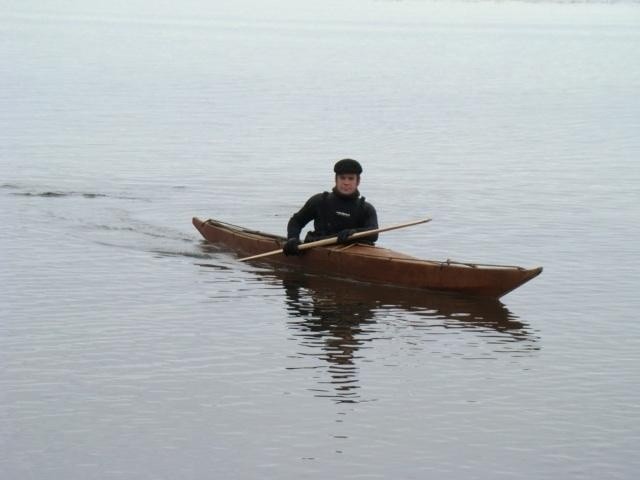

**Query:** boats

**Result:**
xmin=244 ymin=260 xmax=548 ymax=360
xmin=191 ymin=214 xmax=544 ymax=300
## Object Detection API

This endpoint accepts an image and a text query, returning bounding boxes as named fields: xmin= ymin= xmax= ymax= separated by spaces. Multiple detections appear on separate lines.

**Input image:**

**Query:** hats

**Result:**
xmin=335 ymin=159 xmax=362 ymax=175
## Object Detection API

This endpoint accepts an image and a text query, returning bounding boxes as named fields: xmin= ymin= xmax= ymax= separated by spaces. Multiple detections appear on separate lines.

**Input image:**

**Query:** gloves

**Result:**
xmin=337 ymin=228 xmax=356 ymax=243
xmin=284 ymin=238 xmax=305 ymax=258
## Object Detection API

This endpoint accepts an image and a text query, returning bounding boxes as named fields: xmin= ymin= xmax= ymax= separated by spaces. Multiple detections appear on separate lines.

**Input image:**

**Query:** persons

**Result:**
xmin=282 ymin=160 xmax=379 ymax=256
